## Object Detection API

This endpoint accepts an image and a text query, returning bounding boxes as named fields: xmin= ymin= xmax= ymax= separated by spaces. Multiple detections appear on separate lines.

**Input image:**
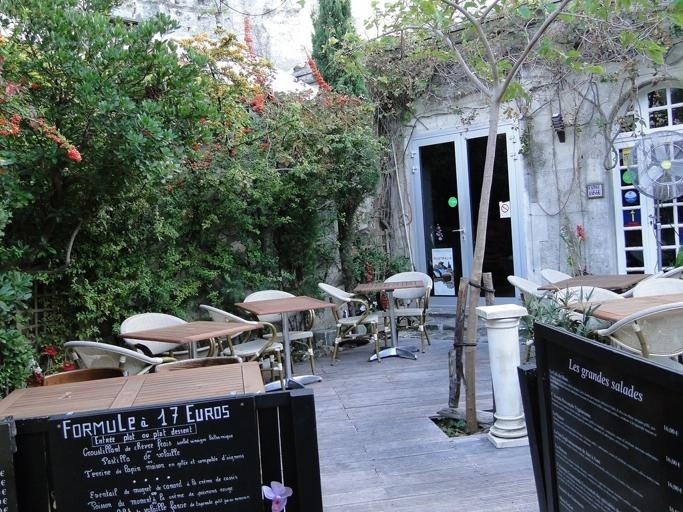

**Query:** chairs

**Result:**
xmin=241 ymin=289 xmax=316 ymax=376
xmin=199 ymin=302 xmax=285 ymax=393
xmin=63 ymin=339 xmax=163 ymax=375
xmin=156 ymin=353 xmax=243 ymax=372
xmin=317 ymin=280 xmax=384 ymax=366
xmin=44 ymin=367 xmax=129 ymax=386
xmin=504 ymin=266 xmax=682 ymax=368
xmin=119 ymin=312 xmax=190 ymax=357
xmin=383 ymin=270 xmax=432 ymax=354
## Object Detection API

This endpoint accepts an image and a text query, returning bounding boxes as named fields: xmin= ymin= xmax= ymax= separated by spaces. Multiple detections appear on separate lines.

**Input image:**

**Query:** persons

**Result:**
xmin=435 ymin=260 xmax=447 ymax=278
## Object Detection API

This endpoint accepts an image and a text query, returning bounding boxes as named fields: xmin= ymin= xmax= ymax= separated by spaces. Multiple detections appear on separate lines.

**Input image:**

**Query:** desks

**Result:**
xmin=234 ymin=296 xmax=337 ymax=391
xmin=353 ymin=280 xmax=425 ymax=361
xmin=1 ymin=361 xmax=268 ymax=422
xmin=120 ymin=319 xmax=265 ymax=359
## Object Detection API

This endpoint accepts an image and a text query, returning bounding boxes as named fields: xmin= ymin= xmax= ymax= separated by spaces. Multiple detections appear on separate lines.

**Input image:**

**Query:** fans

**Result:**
xmin=625 ymin=131 xmax=681 ymax=269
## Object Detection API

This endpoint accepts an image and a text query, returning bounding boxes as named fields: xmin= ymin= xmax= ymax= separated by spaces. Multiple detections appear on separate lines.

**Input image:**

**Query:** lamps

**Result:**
xmin=549 ymin=111 xmax=565 ymax=145
